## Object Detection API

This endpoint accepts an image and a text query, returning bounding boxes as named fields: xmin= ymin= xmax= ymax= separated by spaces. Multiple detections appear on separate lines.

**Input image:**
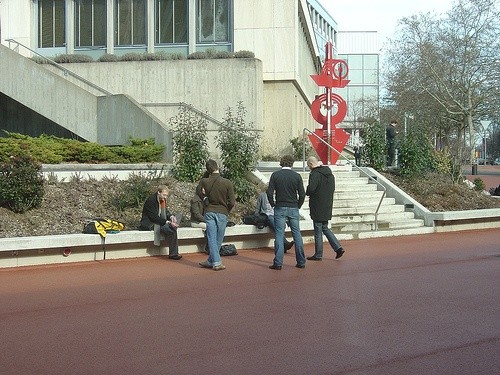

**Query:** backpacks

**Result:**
xmin=243 ymin=213 xmax=268 ymax=229
xmin=82 ymin=218 xmax=124 ymax=237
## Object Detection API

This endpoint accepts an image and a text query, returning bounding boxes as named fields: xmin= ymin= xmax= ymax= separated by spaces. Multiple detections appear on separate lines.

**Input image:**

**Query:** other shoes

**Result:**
xmin=307 ymin=256 xmax=322 ymax=261
xmin=269 ymin=265 xmax=281 ymax=270
xmin=213 ymin=264 xmax=225 ymax=271
xmin=336 ymin=248 xmax=345 ymax=258
xmin=284 ymin=241 xmax=294 ymax=253
xmin=199 ymin=260 xmax=213 ymax=267
xmin=296 ymin=264 xmax=305 ymax=268
xmin=169 ymin=255 xmax=182 ymax=260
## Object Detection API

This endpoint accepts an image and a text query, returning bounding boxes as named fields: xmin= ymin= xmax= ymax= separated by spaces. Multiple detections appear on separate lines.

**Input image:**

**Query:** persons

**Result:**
xmin=140 ymin=185 xmax=182 ymax=260
xmin=353 ymin=142 xmax=361 ymax=166
xmin=387 ymin=120 xmax=398 ymax=165
xmin=256 ymin=182 xmax=296 ymax=253
xmin=306 ymin=157 xmax=347 ymax=260
xmin=267 ymin=156 xmax=307 ymax=270
xmin=199 ymin=159 xmax=235 ymax=269
xmin=192 ymin=183 xmax=222 ymax=255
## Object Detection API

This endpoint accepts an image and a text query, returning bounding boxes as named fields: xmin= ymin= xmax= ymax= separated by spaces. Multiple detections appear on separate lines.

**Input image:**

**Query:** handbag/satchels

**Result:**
xmin=219 ymin=244 xmax=238 ymax=256
